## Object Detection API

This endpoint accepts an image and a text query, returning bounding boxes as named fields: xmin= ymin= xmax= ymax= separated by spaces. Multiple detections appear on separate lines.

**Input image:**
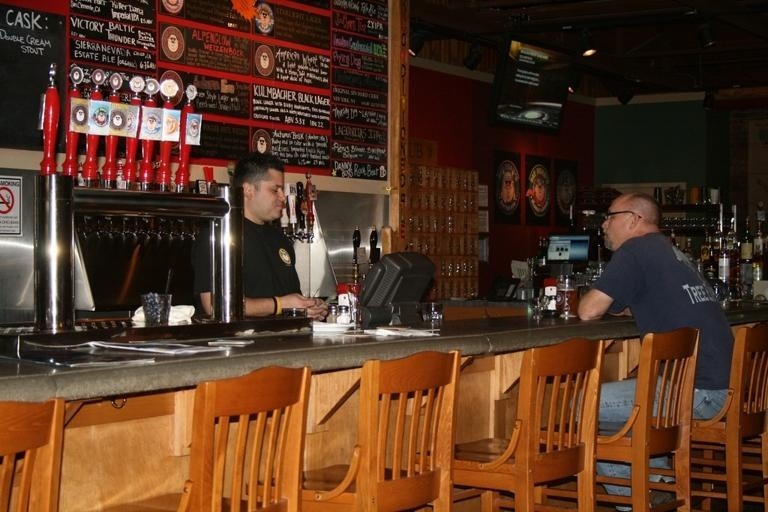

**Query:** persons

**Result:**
xmin=568 ymin=190 xmax=735 ymax=512
xmin=189 ymin=151 xmax=331 ymax=327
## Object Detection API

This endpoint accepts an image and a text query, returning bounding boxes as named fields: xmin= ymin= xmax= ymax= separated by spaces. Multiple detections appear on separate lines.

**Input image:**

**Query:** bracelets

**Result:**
xmin=272 ymin=295 xmax=281 ymax=316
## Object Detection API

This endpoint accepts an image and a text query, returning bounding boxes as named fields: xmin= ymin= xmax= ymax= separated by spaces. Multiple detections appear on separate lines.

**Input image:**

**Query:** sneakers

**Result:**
xmin=648 ymin=480 xmax=674 ymax=508
xmin=665 ymin=480 xmax=677 ymax=500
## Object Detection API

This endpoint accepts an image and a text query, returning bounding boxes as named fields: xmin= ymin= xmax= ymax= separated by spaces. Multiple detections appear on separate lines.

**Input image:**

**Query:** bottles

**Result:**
xmin=680 ymin=237 xmax=693 ymax=259
xmin=716 ymin=238 xmax=731 ymax=284
xmin=740 ymin=216 xmax=753 ymax=263
xmin=753 ymin=219 xmax=764 ymax=261
xmin=698 ymin=227 xmax=713 ymax=264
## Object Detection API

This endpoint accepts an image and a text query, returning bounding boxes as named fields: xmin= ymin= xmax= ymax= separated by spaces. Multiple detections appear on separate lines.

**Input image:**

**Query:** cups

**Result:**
xmin=141 ymin=293 xmax=171 ymax=326
xmin=555 ymin=274 xmax=577 ymax=318
xmin=421 ymin=304 xmax=443 ymax=325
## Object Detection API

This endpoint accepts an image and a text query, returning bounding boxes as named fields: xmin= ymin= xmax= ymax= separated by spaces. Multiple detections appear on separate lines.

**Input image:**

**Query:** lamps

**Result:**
xmin=698 ymin=27 xmax=718 ymax=48
xmin=702 ymin=90 xmax=710 ymax=109
xmin=461 ymin=52 xmax=482 ymax=69
xmin=404 ymin=41 xmax=425 ymax=56
xmin=619 ymin=87 xmax=637 ymax=104
xmin=577 ymin=41 xmax=596 ymax=57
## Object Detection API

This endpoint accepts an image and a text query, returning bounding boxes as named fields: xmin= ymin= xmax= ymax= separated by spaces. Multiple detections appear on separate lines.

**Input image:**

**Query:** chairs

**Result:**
xmin=250 ymin=348 xmax=465 ymax=512
xmin=0 ymin=394 xmax=69 ymax=511
xmin=99 ymin=365 xmax=313 ymax=512
xmin=547 ymin=327 xmax=702 ymax=511
xmin=415 ymin=339 xmax=607 ymax=512
xmin=650 ymin=322 xmax=768 ymax=512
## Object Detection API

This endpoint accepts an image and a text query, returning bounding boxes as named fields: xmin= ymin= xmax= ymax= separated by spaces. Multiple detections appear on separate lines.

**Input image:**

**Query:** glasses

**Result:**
xmin=602 ymin=211 xmax=643 ymax=220
